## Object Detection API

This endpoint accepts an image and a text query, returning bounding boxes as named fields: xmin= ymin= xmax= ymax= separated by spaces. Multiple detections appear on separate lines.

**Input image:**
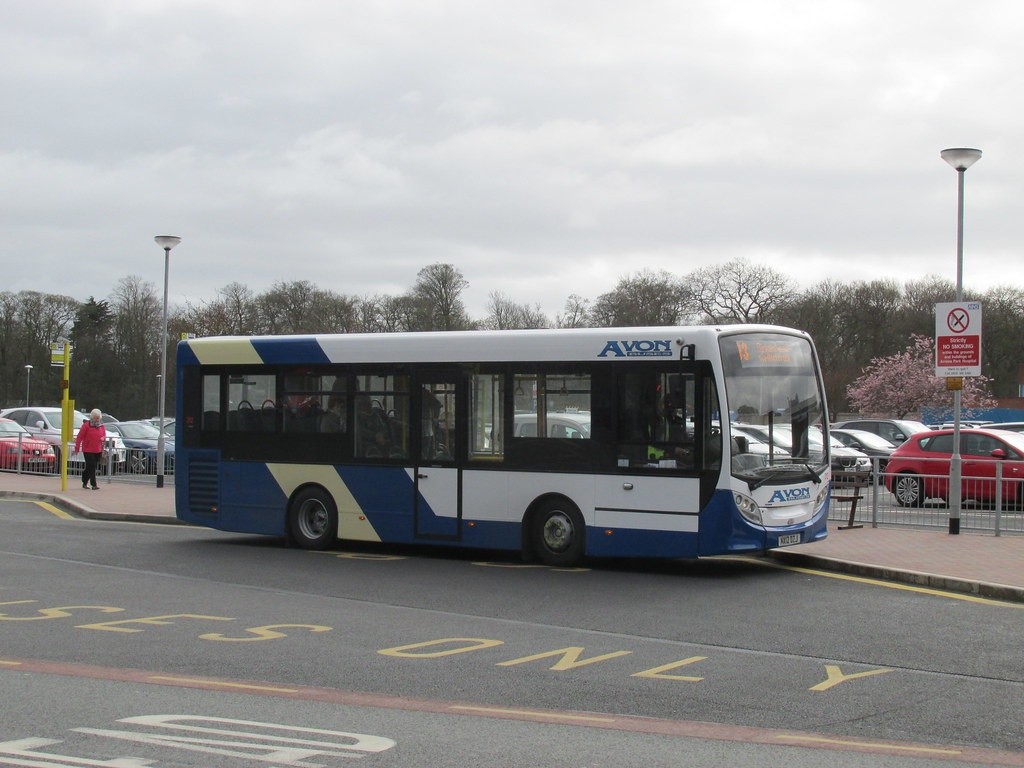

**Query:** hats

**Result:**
xmin=663 ymin=391 xmax=684 ymax=404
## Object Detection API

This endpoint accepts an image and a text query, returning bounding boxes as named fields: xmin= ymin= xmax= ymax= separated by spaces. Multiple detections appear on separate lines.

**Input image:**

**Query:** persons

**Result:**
xmin=648 ymin=398 xmax=694 ymax=461
xmin=75 ymin=408 xmax=107 ymax=490
xmin=318 ymin=375 xmax=442 ymax=464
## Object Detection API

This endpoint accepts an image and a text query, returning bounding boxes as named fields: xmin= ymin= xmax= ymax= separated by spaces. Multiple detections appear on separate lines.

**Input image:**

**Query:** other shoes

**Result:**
xmin=83 ymin=484 xmax=90 ymax=489
xmin=92 ymin=486 xmax=100 ymax=489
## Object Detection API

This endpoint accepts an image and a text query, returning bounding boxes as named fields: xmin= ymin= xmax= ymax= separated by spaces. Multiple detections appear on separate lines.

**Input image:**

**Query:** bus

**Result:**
xmin=176 ymin=323 xmax=830 ymax=569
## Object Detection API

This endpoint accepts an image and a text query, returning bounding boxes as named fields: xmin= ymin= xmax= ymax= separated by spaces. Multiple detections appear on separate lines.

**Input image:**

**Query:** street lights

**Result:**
xmin=24 ymin=365 xmax=34 ymax=406
xmin=940 ymin=147 xmax=983 ymax=535
xmin=154 ymin=236 xmax=181 ymax=488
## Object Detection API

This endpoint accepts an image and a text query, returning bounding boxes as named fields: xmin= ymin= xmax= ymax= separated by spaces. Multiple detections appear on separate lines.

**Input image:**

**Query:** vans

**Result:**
xmin=511 ymin=414 xmax=591 ymax=438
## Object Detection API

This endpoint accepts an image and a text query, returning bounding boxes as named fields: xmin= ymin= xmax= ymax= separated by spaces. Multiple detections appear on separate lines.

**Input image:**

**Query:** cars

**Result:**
xmin=884 ymin=428 xmax=1023 ymax=513
xmin=682 ymin=415 xmax=1024 ymax=487
xmin=0 ymin=407 xmax=177 ymax=475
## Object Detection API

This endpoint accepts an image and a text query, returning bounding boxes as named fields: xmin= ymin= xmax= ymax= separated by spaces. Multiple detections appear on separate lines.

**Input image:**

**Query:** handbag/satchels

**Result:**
xmin=98 ymin=445 xmax=108 ymax=465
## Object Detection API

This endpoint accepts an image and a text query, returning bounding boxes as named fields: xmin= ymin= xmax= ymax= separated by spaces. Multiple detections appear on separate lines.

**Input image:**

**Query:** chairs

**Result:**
xmin=204 ymin=399 xmax=400 ymax=459
xmin=968 ymin=439 xmax=990 ymax=456
